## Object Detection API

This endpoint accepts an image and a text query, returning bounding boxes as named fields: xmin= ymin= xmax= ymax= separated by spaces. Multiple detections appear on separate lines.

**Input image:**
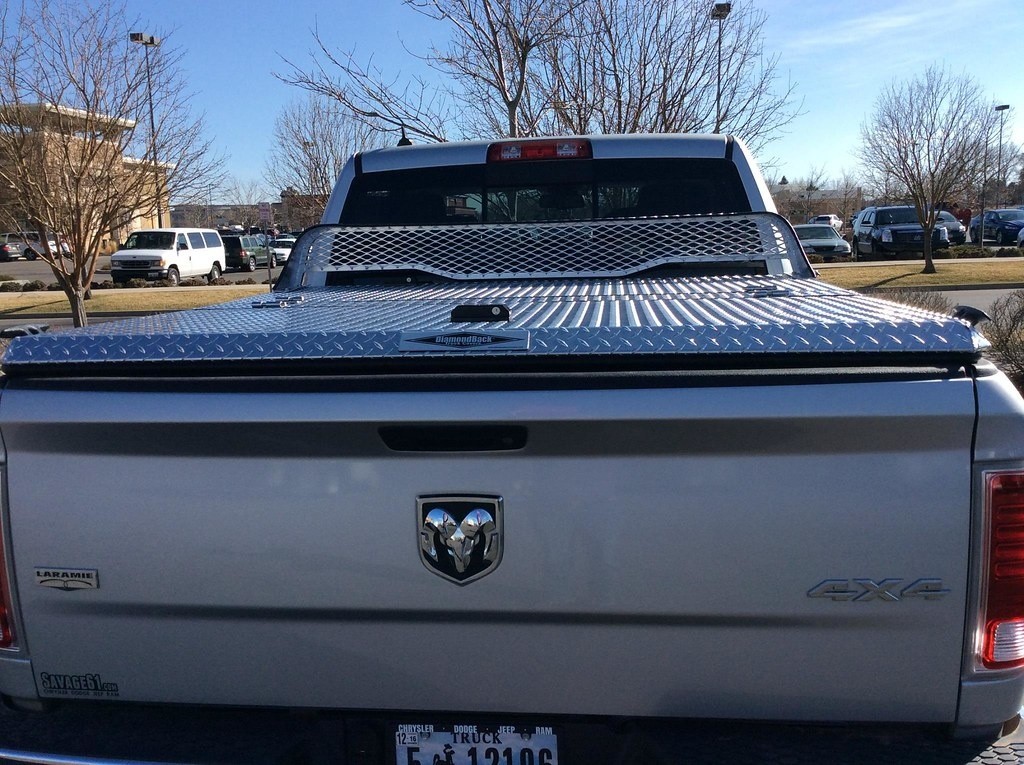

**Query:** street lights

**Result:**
xmin=995 ymin=105 xmax=1009 ymax=209
xmin=131 ymin=31 xmax=163 ymax=230
xmin=209 ymin=183 xmax=214 ymax=227
xmin=711 ymin=3 xmax=732 ymax=132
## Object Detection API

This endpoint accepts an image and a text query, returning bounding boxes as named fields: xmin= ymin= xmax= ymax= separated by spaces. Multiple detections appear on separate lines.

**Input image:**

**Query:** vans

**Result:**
xmin=110 ymin=228 xmax=225 ymax=286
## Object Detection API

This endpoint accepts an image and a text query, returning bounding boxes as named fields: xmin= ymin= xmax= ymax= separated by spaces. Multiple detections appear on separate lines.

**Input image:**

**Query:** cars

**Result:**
xmin=217 ymin=229 xmax=245 ymax=235
xmin=968 ymin=209 xmax=1024 ymax=246
xmin=1017 ymin=228 xmax=1024 ymax=248
xmin=0 ymin=233 xmax=22 ymax=261
xmin=808 ymin=214 xmax=844 ymax=231
xmin=850 ymin=206 xmax=950 ymax=261
xmin=269 ymin=238 xmax=298 ymax=267
xmin=0 ymin=241 xmax=13 ymax=262
xmin=929 ymin=210 xmax=967 ymax=246
xmin=242 ymin=226 xmax=303 ymax=239
xmin=221 ymin=235 xmax=277 ymax=272
xmin=0 ymin=137 xmax=1024 ymax=765
xmin=17 ymin=231 xmax=73 ymax=261
xmin=791 ymin=224 xmax=851 ymax=262
